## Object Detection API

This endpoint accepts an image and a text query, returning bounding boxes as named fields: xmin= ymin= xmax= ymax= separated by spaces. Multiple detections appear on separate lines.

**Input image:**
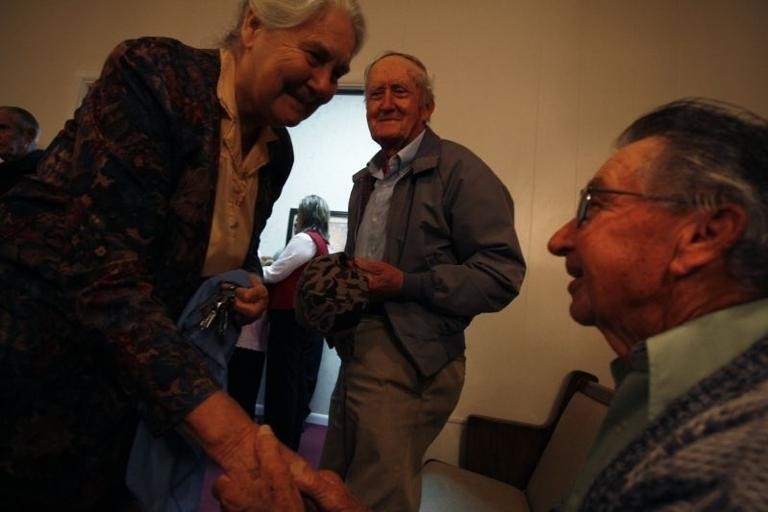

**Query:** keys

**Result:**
xmin=197 ymin=291 xmax=235 ymax=341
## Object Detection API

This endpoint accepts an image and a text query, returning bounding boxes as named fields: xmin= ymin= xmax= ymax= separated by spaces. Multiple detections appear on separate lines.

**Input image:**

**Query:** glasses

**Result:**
xmin=573 ymin=187 xmax=710 ymax=228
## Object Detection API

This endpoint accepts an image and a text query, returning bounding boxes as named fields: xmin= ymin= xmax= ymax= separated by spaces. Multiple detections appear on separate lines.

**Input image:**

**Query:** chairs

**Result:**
xmin=415 ymin=369 xmax=614 ymax=512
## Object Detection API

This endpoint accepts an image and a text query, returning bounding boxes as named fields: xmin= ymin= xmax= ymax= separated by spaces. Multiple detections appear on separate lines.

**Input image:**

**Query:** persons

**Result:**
xmin=0 ymin=105 xmax=50 ymax=196
xmin=320 ymin=51 xmax=528 ymax=511
xmin=210 ymin=94 xmax=766 ymax=512
xmin=259 ymin=193 xmax=331 ymax=454
xmin=226 ymin=298 xmax=268 ymax=425
xmin=0 ymin=2 xmax=366 ymax=511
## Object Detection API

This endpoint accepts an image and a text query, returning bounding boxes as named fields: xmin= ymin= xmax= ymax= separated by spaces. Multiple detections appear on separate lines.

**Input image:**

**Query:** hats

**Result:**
xmin=295 ymin=250 xmax=368 ymax=338
